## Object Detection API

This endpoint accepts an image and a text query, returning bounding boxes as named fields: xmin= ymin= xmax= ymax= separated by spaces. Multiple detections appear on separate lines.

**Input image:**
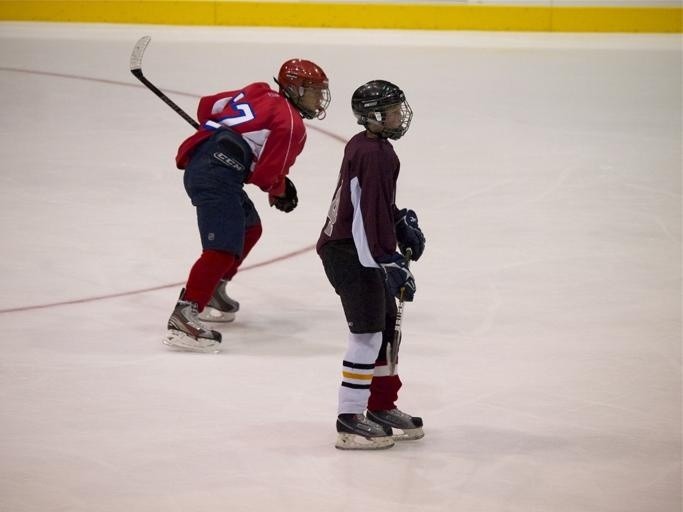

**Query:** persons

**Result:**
xmin=317 ymin=80 xmax=426 ymax=439
xmin=165 ymin=57 xmax=329 ymax=342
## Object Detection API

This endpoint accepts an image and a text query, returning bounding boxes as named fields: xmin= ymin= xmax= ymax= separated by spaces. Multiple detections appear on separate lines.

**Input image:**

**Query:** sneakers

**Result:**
xmin=365 ymin=409 xmax=424 ymax=428
xmin=336 ymin=413 xmax=392 ymax=438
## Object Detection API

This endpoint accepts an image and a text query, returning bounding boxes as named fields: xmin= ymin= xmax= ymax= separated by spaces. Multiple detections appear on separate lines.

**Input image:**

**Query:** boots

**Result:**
xmin=167 ymin=288 xmax=222 ymax=342
xmin=206 ymin=279 xmax=239 ymax=313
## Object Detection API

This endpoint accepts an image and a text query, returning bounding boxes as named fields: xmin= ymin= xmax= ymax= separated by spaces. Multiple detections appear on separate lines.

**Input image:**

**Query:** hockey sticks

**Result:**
xmin=390 ymin=247 xmax=413 ymax=376
xmin=130 ymin=35 xmax=199 ymax=129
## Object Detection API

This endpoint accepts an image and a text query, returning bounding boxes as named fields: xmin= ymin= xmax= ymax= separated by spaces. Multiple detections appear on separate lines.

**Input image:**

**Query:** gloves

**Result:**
xmin=394 ymin=207 xmax=426 ymax=259
xmin=269 ymin=176 xmax=299 ymax=214
xmin=381 ymin=251 xmax=416 ymax=302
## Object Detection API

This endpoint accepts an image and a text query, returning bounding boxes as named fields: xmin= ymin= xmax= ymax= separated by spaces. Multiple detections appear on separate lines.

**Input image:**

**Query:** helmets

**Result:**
xmin=351 ymin=80 xmax=413 ymax=140
xmin=279 ymin=59 xmax=331 ymax=120
